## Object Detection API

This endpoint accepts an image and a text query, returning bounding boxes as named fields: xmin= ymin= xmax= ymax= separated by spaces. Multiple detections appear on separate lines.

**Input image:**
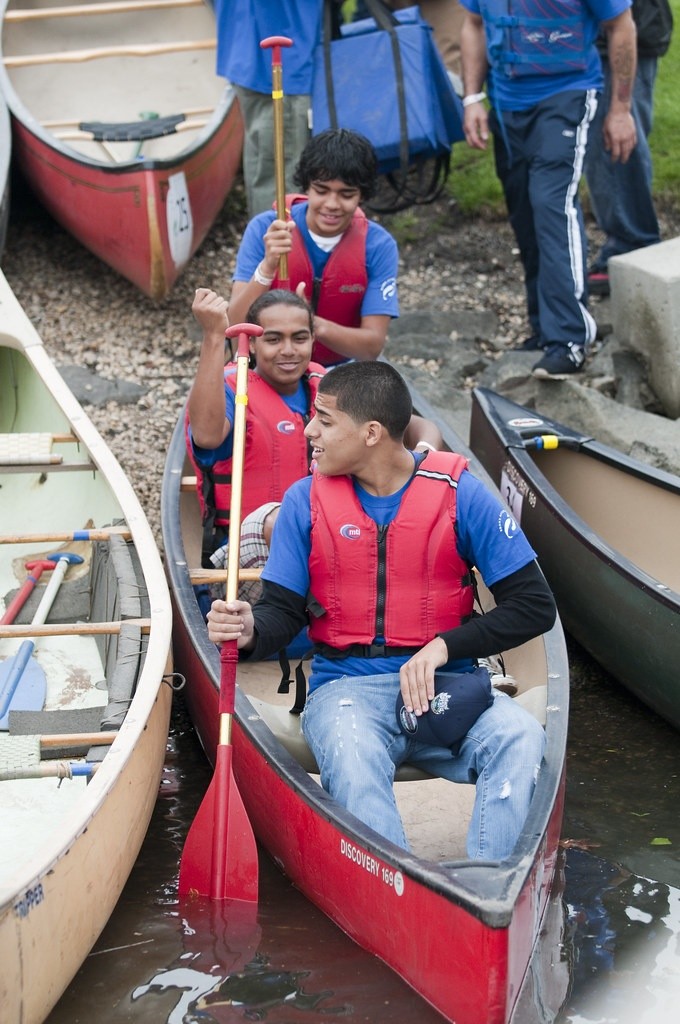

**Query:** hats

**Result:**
xmin=395 ymin=667 xmax=494 ymax=751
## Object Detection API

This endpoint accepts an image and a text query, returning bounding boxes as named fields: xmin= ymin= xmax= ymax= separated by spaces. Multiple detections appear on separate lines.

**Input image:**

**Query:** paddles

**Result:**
xmin=1 ymin=553 xmax=84 ymax=718
xmin=175 ymin=320 xmax=264 ymax=938
xmin=258 ymin=34 xmax=293 ymax=291
xmin=0 ymin=560 xmax=56 ymax=625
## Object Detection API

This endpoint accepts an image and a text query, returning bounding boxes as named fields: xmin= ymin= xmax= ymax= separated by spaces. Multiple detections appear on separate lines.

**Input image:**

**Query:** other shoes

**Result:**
xmin=587 ymin=263 xmax=609 ymax=296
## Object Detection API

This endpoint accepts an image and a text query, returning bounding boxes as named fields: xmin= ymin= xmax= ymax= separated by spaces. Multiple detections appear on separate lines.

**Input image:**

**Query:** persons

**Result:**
xmin=207 ymin=359 xmax=559 ymax=866
xmin=225 ymin=126 xmax=399 ymax=371
xmin=583 ymin=0 xmax=664 ymax=295
xmin=212 ymin=0 xmax=327 ymax=232
xmin=460 ymin=0 xmax=638 ymax=379
xmin=188 ymin=287 xmax=519 ymax=698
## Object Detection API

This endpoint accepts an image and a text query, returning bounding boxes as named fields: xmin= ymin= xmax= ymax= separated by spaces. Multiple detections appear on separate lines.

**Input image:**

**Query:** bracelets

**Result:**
xmin=417 ymin=441 xmax=438 ymax=453
xmin=255 ymin=268 xmax=274 ymax=287
xmin=462 ymin=91 xmax=487 ymax=107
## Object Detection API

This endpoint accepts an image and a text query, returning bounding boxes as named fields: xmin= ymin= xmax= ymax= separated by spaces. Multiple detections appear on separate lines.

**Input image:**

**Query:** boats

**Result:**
xmin=161 ymin=354 xmax=574 ymax=1024
xmin=2 ymin=0 xmax=243 ymax=305
xmin=464 ymin=379 xmax=680 ymax=728
xmin=0 ymin=259 xmax=186 ymax=1023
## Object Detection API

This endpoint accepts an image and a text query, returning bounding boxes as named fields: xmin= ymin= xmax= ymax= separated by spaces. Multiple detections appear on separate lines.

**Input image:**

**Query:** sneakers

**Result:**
xmin=523 ymin=335 xmax=545 ymax=351
xmin=478 ymin=658 xmax=517 ymax=697
xmin=531 ymin=342 xmax=585 ymax=379
